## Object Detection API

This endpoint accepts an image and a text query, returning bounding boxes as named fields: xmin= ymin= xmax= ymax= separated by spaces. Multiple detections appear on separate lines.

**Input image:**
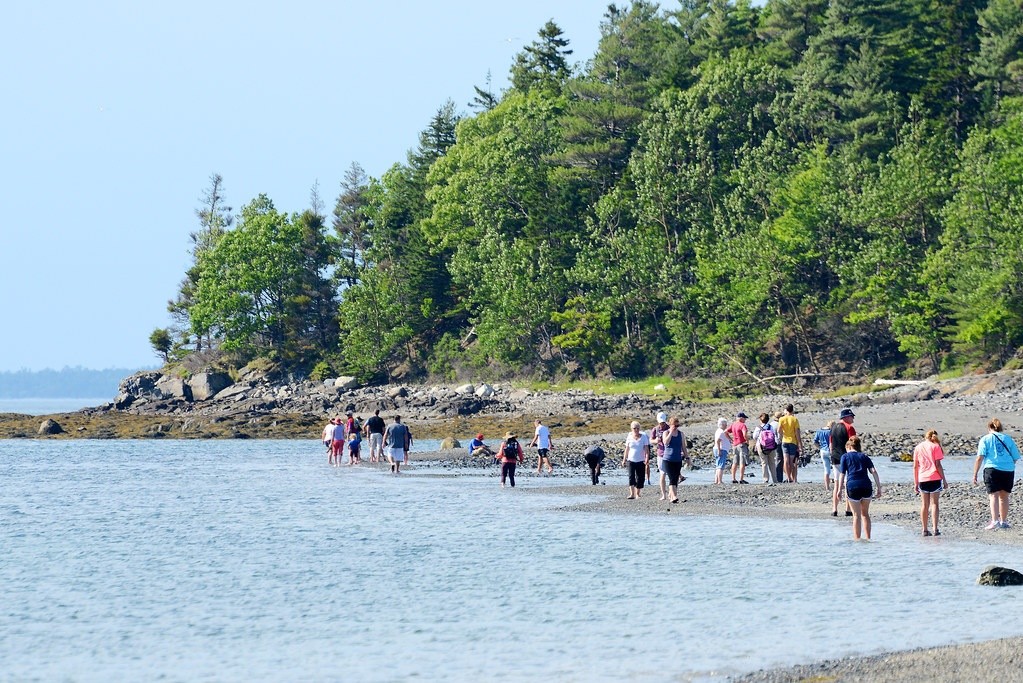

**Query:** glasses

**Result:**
xmin=633 ymin=428 xmax=640 ymax=429
xmin=674 ymin=424 xmax=679 ymax=428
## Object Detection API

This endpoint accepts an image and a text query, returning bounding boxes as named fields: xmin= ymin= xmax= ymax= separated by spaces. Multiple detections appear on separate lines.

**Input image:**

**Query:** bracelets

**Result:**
xmin=686 ymin=457 xmax=690 ymax=460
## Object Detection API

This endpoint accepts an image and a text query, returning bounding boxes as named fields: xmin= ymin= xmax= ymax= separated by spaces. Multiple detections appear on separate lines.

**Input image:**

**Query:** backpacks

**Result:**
xmin=504 ymin=439 xmax=518 ymax=460
xmin=758 ymin=424 xmax=775 ymax=451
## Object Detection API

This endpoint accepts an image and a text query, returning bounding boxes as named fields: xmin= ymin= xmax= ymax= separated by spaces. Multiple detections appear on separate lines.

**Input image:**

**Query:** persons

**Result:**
xmin=530 ymin=419 xmax=553 ymax=473
xmin=913 ymin=430 xmax=948 ymax=536
xmin=724 ymin=412 xmax=749 ymax=484
xmin=973 ymin=418 xmax=1021 ymax=530
xmin=468 ymin=434 xmax=496 ymax=456
xmin=650 ymin=412 xmax=686 ymax=500
xmin=622 ymin=421 xmax=649 ymax=498
xmin=753 ymin=403 xmax=805 ymax=484
xmin=836 ymin=436 xmax=882 ymax=539
xmin=495 ymin=431 xmax=524 ymax=487
xmin=441 ymin=433 xmax=461 ymax=450
xmin=322 ymin=409 xmax=414 ymax=473
xmin=584 ymin=445 xmax=605 ymax=485
xmin=814 ymin=420 xmax=836 ymax=489
xmin=662 ymin=416 xmax=691 ymax=502
xmin=829 ymin=409 xmax=856 ymax=517
xmin=712 ymin=418 xmax=730 ymax=483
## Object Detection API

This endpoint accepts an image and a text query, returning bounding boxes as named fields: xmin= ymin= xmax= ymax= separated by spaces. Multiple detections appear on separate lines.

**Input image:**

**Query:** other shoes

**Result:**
xmin=984 ymin=521 xmax=1000 ymax=530
xmin=732 ymin=479 xmax=739 ymax=483
xmin=830 ymin=511 xmax=837 ymax=517
xmin=486 ymin=451 xmax=497 ymax=457
xmin=1001 ymin=522 xmax=1011 ymax=529
xmin=740 ymin=480 xmax=749 ymax=484
xmin=923 ymin=530 xmax=932 ymax=536
xmin=679 ymin=477 xmax=686 ymax=483
xmin=845 ymin=510 xmax=852 ymax=516
xmin=934 ymin=529 xmax=941 ymax=536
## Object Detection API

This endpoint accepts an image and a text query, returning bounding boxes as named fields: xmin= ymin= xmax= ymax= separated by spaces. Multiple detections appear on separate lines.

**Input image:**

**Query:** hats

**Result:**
xmin=332 ymin=418 xmax=344 ymax=425
xmin=476 ymin=434 xmax=483 ymax=440
xmin=345 ymin=411 xmax=353 ymax=416
xmin=657 ymin=412 xmax=666 ymax=423
xmin=502 ymin=432 xmax=518 ymax=439
xmin=738 ymin=413 xmax=749 ymax=418
xmin=840 ymin=409 xmax=855 ymax=420
